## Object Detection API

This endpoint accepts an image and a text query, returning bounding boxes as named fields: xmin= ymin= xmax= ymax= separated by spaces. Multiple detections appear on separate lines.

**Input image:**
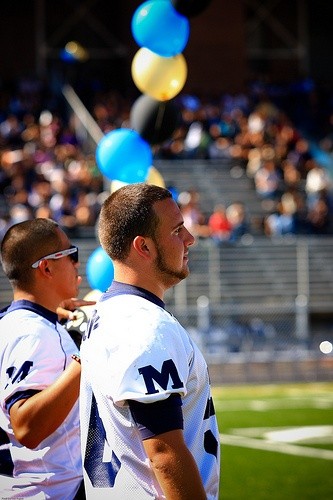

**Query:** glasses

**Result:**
xmin=31 ymin=245 xmax=80 ymax=268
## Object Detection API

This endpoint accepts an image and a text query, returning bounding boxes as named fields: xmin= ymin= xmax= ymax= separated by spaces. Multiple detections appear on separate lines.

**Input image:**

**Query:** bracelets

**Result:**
xmin=72 ymin=354 xmax=81 ymax=364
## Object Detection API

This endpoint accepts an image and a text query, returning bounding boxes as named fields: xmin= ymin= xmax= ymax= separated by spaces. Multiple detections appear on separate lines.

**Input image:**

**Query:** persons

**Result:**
xmin=79 ymin=183 xmax=218 ymax=500
xmin=0 ymin=217 xmax=97 ymax=500
xmin=0 ymin=40 xmax=333 ymax=249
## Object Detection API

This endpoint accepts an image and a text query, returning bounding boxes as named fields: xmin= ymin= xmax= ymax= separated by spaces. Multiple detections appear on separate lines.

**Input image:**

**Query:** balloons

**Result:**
xmin=86 ymin=247 xmax=114 ymax=293
xmin=130 ymin=0 xmax=190 ymax=55
xmin=130 ymin=94 xmax=184 ymax=147
xmin=97 ymin=128 xmax=152 ymax=185
xmin=109 ymin=165 xmax=167 ymax=200
xmin=130 ymin=46 xmax=187 ymax=100
